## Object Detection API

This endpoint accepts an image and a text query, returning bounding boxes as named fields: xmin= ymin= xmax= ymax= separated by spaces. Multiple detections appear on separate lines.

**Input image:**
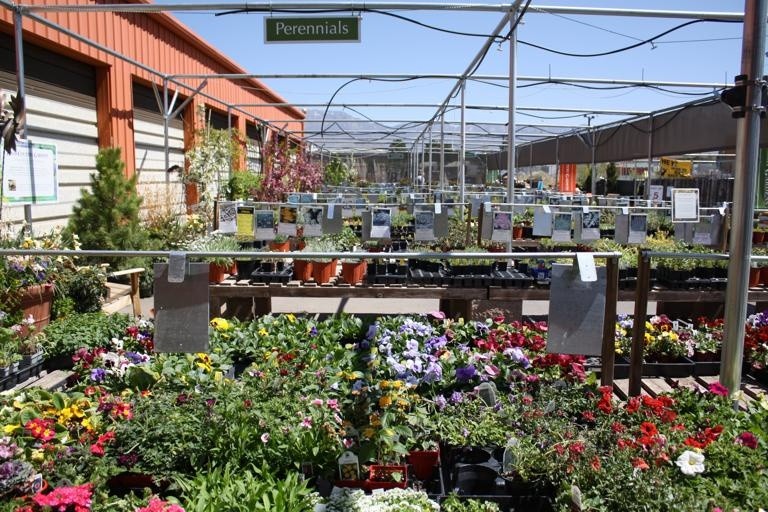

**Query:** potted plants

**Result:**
xmin=605 ymin=382 xmax=768 ymax=512
xmin=604 ymin=213 xmax=768 ymax=289
xmin=609 ymin=306 xmax=767 ymax=388
xmin=0 ymin=217 xmax=607 ymax=512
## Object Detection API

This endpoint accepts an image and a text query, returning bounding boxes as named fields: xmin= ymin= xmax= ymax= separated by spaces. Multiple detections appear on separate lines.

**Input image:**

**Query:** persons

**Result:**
xmin=413 ymin=169 xmax=426 ymax=192
xmin=574 ymin=183 xmax=582 ymax=194
xmin=502 ymin=173 xmax=509 ymax=188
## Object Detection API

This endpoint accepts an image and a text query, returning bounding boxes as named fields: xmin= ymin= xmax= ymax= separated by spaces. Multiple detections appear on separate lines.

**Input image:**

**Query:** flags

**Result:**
xmin=556 ymin=164 xmax=579 ymax=193
